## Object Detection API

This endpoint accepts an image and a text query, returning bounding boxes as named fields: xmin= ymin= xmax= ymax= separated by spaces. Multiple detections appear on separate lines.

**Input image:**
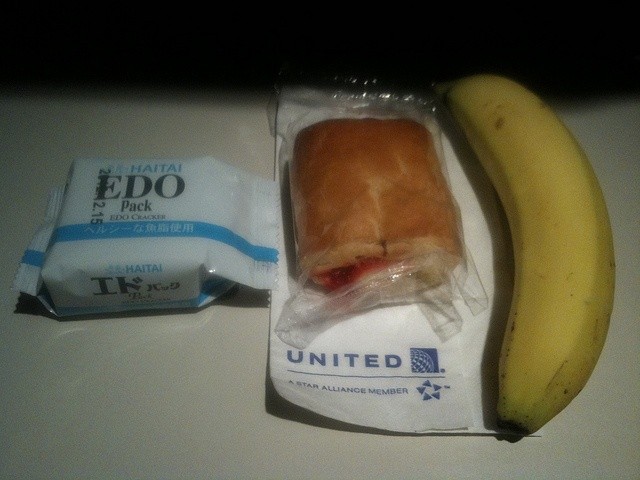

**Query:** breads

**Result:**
xmin=290 ymin=117 xmax=463 ymax=301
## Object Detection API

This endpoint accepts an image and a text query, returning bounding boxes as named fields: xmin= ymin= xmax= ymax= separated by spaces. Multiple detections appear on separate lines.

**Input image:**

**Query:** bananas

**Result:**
xmin=444 ymin=74 xmax=616 ymax=435
